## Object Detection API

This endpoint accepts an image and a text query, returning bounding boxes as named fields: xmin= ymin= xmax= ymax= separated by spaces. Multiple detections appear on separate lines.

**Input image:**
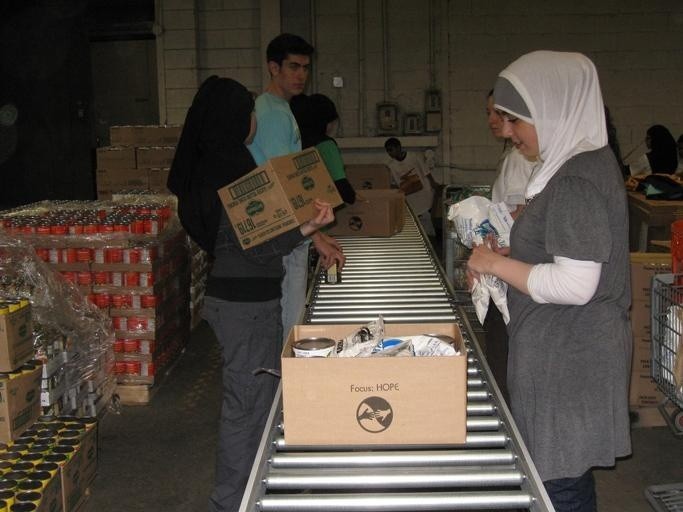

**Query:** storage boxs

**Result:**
xmin=324 ymin=187 xmax=407 ymax=238
xmin=280 ymin=322 xmax=467 ymax=450
xmin=626 ymin=252 xmax=678 ymax=423
xmin=212 ymin=147 xmax=346 ymax=249
xmin=0 ymin=295 xmax=115 ymax=509
xmin=90 ymin=120 xmax=212 ymax=200
xmin=342 ymin=162 xmax=393 ymax=190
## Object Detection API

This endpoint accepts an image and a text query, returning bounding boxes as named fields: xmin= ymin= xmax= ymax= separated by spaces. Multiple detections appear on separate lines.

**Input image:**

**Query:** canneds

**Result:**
xmin=423 ymin=333 xmax=456 ymax=350
xmin=325 ymin=260 xmax=341 ymax=285
xmin=1 ymin=294 xmax=115 ymax=512
xmin=2 ymin=187 xmax=191 ymax=377
xmin=291 ymin=336 xmax=335 ymax=358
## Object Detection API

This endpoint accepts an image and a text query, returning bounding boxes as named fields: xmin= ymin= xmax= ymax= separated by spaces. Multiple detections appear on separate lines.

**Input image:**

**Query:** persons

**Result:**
xmin=602 ymin=106 xmax=624 ymax=177
xmin=483 ymin=87 xmax=535 ymax=412
xmin=244 ymin=33 xmax=313 ymax=346
xmin=166 ymin=74 xmax=346 ymax=512
xmin=292 ymin=93 xmax=361 ymax=204
xmin=463 ymin=48 xmax=633 ymax=510
xmin=384 ymin=137 xmax=441 ymax=217
xmin=381 ymin=110 xmax=393 ymax=126
xmin=624 ymin=126 xmax=682 ymax=177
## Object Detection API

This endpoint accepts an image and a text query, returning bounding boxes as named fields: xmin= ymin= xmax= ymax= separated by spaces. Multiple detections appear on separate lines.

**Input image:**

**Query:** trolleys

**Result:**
xmin=438 ymin=183 xmax=492 ymax=332
xmin=647 ymin=270 xmax=682 ymax=442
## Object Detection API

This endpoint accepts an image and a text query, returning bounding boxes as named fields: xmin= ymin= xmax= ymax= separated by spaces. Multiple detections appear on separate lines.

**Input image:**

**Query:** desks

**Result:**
xmin=624 ymin=190 xmax=682 ymax=253
